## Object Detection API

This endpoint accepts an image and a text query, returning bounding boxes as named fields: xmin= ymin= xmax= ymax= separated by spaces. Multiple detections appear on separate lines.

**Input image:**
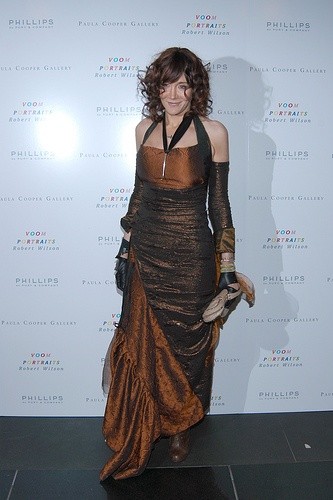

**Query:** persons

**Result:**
xmin=98 ymin=47 xmax=256 ymax=488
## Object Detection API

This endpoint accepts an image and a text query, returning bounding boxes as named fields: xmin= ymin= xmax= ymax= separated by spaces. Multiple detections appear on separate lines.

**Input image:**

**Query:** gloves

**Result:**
xmin=213 ymin=271 xmax=239 ymax=309
xmin=115 ymin=256 xmax=128 ymax=292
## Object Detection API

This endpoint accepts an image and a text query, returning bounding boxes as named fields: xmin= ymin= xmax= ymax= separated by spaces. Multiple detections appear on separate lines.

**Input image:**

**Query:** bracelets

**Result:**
xmin=218 ymin=252 xmax=235 ymax=263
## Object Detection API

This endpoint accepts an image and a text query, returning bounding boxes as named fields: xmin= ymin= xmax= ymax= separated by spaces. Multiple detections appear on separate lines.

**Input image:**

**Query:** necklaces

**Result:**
xmin=161 ymin=111 xmax=193 ymax=182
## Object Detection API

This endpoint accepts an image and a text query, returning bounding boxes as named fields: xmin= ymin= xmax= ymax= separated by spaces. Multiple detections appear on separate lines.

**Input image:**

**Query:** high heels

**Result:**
xmin=167 ymin=427 xmax=190 ymax=464
